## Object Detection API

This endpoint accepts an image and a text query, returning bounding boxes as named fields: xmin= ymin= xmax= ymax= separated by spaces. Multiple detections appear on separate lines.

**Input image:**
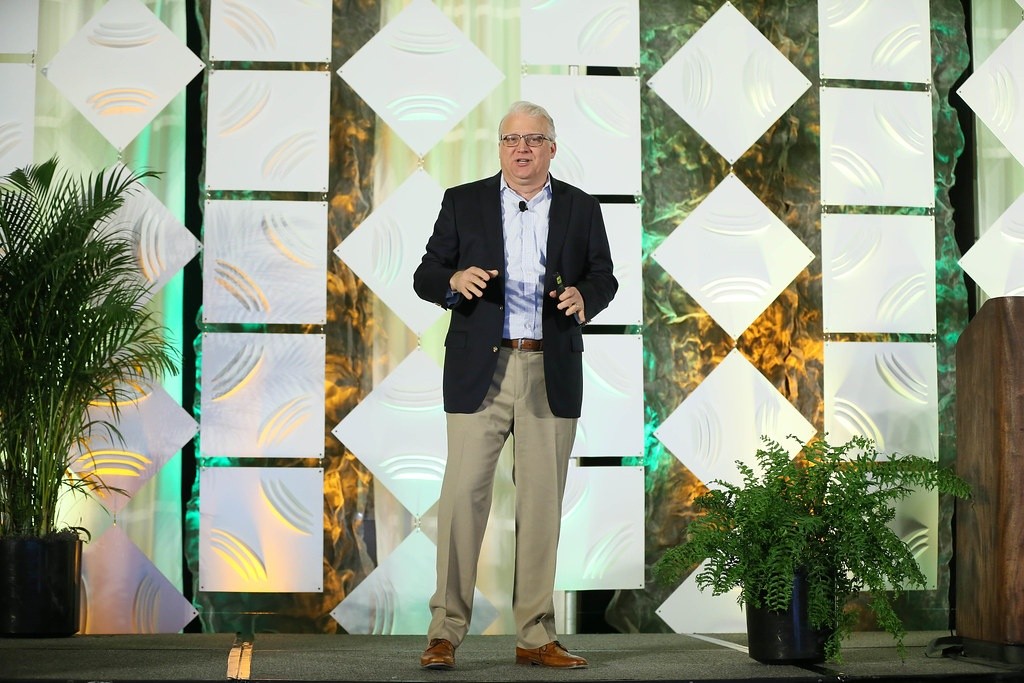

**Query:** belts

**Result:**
xmin=500 ymin=337 xmax=544 ymax=352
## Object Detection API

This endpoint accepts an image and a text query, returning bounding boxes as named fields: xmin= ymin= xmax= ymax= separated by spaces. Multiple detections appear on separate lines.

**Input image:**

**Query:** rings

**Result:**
xmin=575 ymin=302 xmax=580 ymax=310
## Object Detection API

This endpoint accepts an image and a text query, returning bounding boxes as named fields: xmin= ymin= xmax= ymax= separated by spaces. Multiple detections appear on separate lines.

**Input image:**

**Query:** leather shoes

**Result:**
xmin=515 ymin=641 xmax=588 ymax=670
xmin=420 ymin=639 xmax=455 ymax=669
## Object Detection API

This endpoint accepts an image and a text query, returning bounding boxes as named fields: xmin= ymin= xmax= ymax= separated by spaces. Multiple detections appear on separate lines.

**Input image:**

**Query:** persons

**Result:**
xmin=414 ymin=104 xmax=619 ymax=669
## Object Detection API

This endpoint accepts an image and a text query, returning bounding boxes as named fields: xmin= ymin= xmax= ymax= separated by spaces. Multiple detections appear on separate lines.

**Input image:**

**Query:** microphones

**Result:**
xmin=552 ymin=271 xmax=585 ymax=325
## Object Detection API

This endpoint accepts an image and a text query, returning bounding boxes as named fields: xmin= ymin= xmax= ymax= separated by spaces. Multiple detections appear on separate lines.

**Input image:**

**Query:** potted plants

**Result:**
xmin=652 ymin=433 xmax=976 ymax=666
xmin=0 ymin=149 xmax=182 ymax=643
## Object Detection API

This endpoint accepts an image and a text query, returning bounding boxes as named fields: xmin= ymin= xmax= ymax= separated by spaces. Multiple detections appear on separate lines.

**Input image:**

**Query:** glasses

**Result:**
xmin=500 ymin=134 xmax=552 ymax=147
